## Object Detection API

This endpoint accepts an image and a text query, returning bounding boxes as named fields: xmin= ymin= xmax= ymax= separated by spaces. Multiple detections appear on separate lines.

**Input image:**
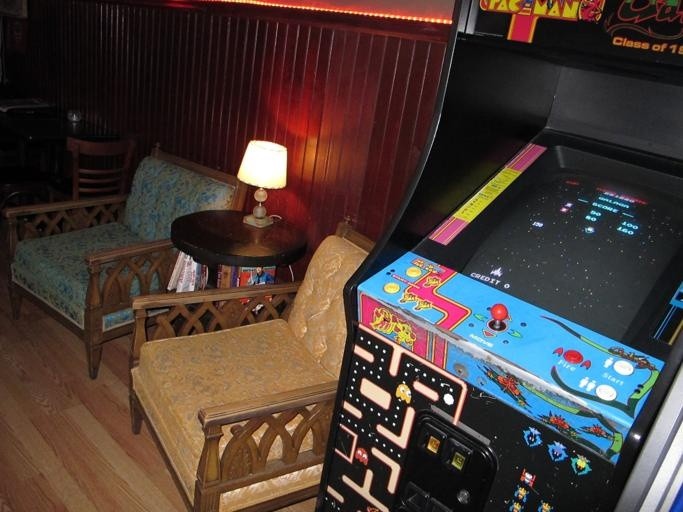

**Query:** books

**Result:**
xmin=164 ymin=250 xmax=276 ymax=313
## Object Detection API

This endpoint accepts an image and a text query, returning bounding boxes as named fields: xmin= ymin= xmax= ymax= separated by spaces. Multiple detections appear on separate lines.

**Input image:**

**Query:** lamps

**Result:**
xmin=236 ymin=140 xmax=288 ymax=228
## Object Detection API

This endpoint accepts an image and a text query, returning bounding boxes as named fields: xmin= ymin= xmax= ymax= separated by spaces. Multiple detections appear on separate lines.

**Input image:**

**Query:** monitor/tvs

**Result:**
xmin=458 ymin=145 xmax=682 ymax=344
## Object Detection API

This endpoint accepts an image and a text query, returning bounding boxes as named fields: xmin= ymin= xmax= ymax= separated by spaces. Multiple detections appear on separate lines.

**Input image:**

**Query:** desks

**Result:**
xmin=171 ymin=210 xmax=307 ymax=336
xmin=0 ymin=98 xmax=68 ymax=184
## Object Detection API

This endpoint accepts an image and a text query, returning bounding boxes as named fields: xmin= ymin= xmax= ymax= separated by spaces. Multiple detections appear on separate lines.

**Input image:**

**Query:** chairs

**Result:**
xmin=44 ymin=134 xmax=137 ymax=204
xmin=2 ymin=148 xmax=247 ymax=381
xmin=129 ymin=221 xmax=377 ymax=512
